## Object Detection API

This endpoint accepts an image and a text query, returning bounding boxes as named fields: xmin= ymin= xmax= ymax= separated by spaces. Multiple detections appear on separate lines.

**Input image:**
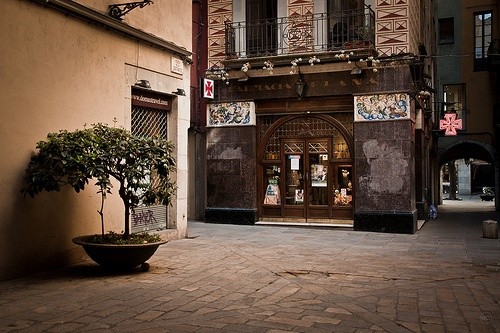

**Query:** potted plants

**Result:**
xmin=19 ymin=123 xmax=179 ymax=269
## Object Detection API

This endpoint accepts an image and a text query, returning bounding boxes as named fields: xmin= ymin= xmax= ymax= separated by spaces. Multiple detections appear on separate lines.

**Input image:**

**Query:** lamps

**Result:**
xmin=294 ymin=74 xmax=307 ymax=100
